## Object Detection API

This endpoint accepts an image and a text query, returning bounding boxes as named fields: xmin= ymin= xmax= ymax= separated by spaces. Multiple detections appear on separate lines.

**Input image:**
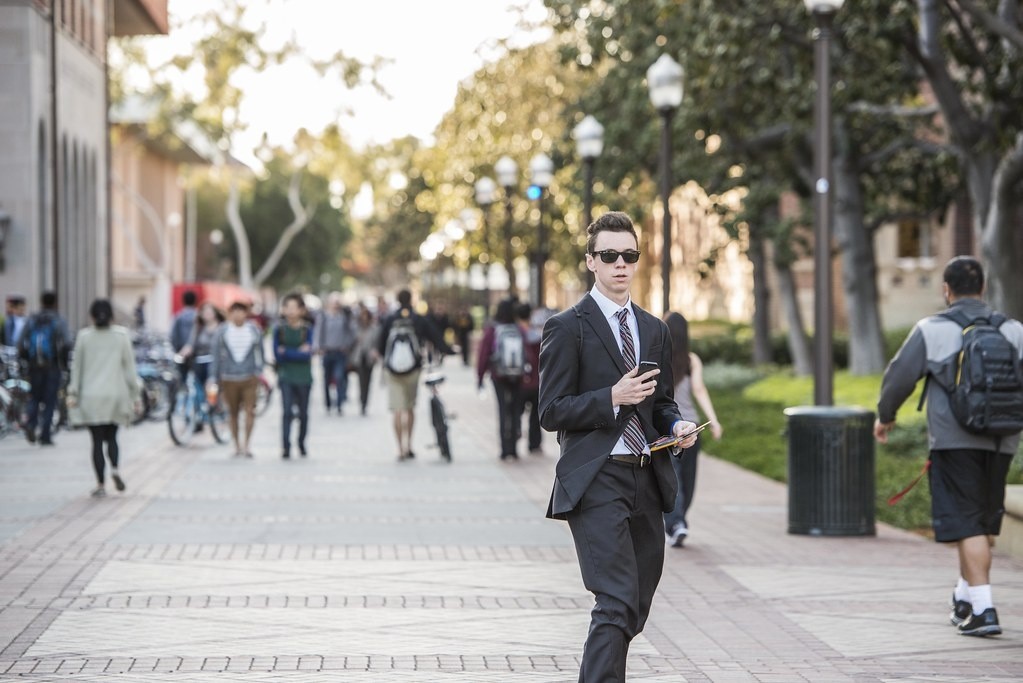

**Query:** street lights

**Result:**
xmin=800 ymin=0 xmax=846 ymax=406
xmin=496 ymin=154 xmax=519 ymax=297
xmin=473 ymin=174 xmax=494 ymax=327
xmin=647 ymin=52 xmax=687 ymax=311
xmin=529 ymin=151 xmax=553 ymax=306
xmin=571 ymin=113 xmax=606 ymax=292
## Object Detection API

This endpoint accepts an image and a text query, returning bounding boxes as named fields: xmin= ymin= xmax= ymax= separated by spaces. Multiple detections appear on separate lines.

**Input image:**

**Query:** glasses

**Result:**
xmin=591 ymin=248 xmax=641 ymax=264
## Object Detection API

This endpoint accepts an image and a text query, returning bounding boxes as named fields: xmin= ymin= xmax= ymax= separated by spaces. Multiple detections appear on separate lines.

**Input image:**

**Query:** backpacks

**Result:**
xmin=383 ymin=311 xmax=424 ymax=375
xmin=487 ymin=316 xmax=524 ymax=381
xmin=916 ymin=308 xmax=1023 ymax=433
xmin=23 ymin=310 xmax=61 ymax=373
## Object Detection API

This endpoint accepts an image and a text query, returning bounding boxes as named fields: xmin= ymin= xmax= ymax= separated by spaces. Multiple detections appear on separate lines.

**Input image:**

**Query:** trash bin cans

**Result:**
xmin=782 ymin=407 xmax=876 ymax=536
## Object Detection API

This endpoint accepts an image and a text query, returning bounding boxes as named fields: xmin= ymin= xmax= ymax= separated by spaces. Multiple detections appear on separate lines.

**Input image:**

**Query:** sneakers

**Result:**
xmin=958 ymin=607 xmax=1002 ymax=636
xmin=669 ymin=522 xmax=688 ymax=546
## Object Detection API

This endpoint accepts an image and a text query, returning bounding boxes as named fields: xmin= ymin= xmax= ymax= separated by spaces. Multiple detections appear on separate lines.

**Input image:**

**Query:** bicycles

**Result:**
xmin=419 ymin=353 xmax=456 ymax=462
xmin=1 ymin=336 xmax=271 ymax=446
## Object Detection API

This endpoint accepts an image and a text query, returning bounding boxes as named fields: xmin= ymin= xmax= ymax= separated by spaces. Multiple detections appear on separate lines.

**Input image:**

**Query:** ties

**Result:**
xmin=613 ymin=308 xmax=646 ymax=457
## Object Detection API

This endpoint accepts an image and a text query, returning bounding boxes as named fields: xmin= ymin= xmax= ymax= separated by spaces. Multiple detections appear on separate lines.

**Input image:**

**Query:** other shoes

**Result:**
xmin=91 ymin=487 xmax=106 ymax=496
xmin=20 ymin=420 xmax=34 ymax=443
xmin=111 ymin=470 xmax=125 ymax=491
xmin=39 ymin=430 xmax=52 ymax=445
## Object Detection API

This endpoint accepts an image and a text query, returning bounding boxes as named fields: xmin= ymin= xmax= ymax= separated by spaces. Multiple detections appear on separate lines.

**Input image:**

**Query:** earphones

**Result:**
xmin=944 ymin=290 xmax=948 ymax=298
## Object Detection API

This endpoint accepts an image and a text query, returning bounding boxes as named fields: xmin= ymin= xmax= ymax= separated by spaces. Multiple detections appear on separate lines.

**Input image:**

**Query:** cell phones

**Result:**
xmin=636 ymin=361 xmax=658 ymax=383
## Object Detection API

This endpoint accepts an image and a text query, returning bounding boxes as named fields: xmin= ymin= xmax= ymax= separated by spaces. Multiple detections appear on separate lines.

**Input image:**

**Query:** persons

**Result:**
xmin=538 ymin=210 xmax=697 ymax=683
xmin=873 ymin=255 xmax=1023 ymax=636
xmin=0 ymin=285 xmax=574 ymax=498
xmin=660 ymin=312 xmax=723 ymax=548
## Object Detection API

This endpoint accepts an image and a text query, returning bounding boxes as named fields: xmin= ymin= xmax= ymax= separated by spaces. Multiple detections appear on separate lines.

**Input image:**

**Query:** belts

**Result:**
xmin=608 ymin=454 xmax=650 ymax=468
